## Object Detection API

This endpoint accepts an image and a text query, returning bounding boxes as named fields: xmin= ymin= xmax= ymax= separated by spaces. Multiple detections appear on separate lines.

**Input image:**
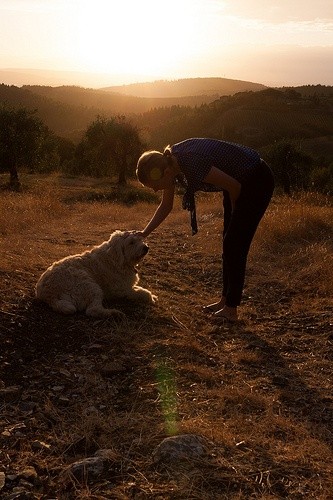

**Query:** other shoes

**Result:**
xmin=209 ymin=309 xmax=238 ymax=321
xmin=201 ymin=301 xmax=224 ymax=312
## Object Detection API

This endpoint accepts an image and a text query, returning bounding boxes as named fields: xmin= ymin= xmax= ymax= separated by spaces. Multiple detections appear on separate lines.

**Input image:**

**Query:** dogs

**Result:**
xmin=35 ymin=229 xmax=158 ymax=318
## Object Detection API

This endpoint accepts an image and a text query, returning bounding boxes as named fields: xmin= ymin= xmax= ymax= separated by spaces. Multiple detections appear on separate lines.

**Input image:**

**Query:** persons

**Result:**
xmin=136 ymin=138 xmax=274 ymax=322
